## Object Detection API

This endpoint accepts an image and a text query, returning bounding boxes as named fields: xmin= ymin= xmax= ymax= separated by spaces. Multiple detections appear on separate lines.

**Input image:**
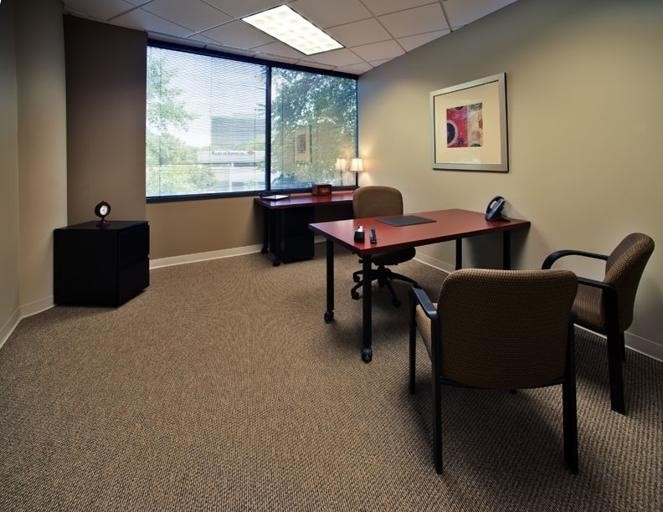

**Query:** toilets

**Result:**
xmin=485 ymin=195 xmax=511 ymax=224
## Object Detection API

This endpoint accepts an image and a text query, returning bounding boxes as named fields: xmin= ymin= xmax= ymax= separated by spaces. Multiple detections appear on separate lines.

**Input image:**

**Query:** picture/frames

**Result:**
xmin=254 ymin=189 xmax=355 ymax=266
xmin=307 ymin=207 xmax=531 ymax=364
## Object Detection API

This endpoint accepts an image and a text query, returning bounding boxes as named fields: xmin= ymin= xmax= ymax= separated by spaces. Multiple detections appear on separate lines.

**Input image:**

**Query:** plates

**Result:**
xmin=52 ymin=219 xmax=150 ymax=310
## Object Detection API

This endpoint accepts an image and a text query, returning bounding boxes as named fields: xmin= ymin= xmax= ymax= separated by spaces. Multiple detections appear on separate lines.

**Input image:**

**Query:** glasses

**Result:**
xmin=348 ymin=159 xmax=366 ymax=188
xmin=336 ymin=157 xmax=347 ymax=186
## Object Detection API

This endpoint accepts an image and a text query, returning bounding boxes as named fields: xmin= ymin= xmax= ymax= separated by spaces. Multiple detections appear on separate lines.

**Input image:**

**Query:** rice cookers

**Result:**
xmin=369 ymin=223 xmax=377 ymax=244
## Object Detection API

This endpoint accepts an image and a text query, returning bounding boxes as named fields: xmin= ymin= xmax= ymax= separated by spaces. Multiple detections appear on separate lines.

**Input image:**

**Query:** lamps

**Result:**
xmin=409 ymin=268 xmax=580 ymax=475
xmin=350 ymin=185 xmax=425 ymax=300
xmin=540 ymin=231 xmax=655 ymax=415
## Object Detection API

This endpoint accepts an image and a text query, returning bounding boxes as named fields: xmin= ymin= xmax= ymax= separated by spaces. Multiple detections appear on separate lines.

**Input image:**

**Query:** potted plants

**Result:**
xmin=292 ymin=125 xmax=314 ymax=163
xmin=428 ymin=70 xmax=511 ymax=175
